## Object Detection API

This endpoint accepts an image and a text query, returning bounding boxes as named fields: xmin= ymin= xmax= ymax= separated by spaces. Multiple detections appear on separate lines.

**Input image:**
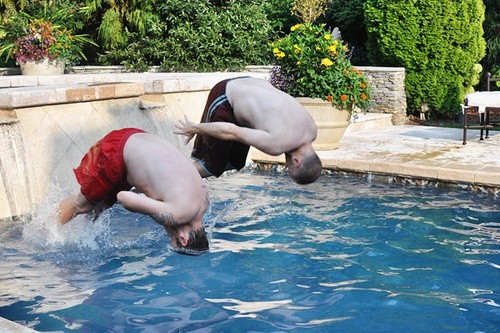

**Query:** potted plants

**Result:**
xmin=268 ymin=22 xmax=373 ymax=150
xmin=0 ymin=10 xmax=99 ymax=75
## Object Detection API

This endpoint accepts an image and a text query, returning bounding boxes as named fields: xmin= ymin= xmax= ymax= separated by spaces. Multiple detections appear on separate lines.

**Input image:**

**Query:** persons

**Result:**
xmin=174 ymin=75 xmax=322 ymax=185
xmin=47 ymin=128 xmax=209 ymax=256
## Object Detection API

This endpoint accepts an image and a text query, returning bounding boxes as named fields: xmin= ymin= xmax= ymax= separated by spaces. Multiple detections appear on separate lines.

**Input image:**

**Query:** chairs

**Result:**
xmin=459 ymin=71 xmax=500 ymax=146
